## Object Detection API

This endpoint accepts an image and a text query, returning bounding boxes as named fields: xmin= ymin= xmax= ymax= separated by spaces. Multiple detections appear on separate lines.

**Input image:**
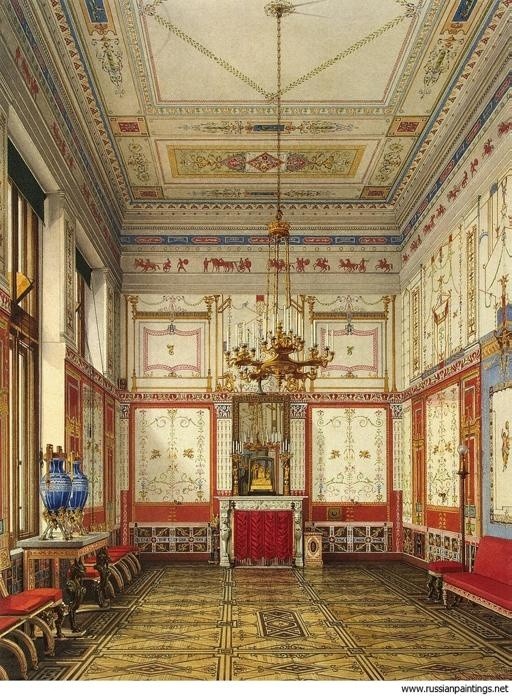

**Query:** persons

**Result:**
xmin=238 ymin=258 xmax=251 ymax=273
xmin=203 ymin=257 xmax=210 ymax=273
xmin=163 ymin=258 xmax=171 ymax=273
xmin=177 ymin=258 xmax=187 ymax=273
xmin=270 ymin=258 xmax=305 ymax=273
xmin=382 ymin=258 xmax=386 ymax=265
xmin=360 ymin=258 xmax=365 ymax=267
xmin=322 ymin=258 xmax=329 ymax=263
xmin=141 ymin=258 xmax=150 ymax=272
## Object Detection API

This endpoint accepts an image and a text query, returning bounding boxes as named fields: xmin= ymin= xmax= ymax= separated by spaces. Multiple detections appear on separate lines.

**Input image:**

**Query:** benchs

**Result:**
xmin=443 ymin=536 xmax=512 ymax=619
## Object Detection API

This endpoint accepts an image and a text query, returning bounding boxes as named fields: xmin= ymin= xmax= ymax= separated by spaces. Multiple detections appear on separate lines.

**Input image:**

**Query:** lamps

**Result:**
xmin=225 ymin=0 xmax=335 ymax=400
xmin=456 ymin=445 xmax=469 ymax=480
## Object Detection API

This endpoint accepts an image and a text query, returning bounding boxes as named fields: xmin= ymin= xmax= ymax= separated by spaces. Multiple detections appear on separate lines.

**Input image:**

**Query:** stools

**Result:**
xmin=427 ymin=561 xmax=464 ymax=603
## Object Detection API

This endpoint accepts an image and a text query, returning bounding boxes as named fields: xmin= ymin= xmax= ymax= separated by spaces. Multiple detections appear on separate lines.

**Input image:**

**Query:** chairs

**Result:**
xmin=0 ymin=523 xmax=143 ymax=680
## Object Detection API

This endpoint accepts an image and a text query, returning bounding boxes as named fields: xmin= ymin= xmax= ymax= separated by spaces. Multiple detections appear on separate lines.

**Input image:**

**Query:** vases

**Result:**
xmin=41 ymin=444 xmax=73 ymax=541
xmin=71 ymin=460 xmax=89 ymax=536
